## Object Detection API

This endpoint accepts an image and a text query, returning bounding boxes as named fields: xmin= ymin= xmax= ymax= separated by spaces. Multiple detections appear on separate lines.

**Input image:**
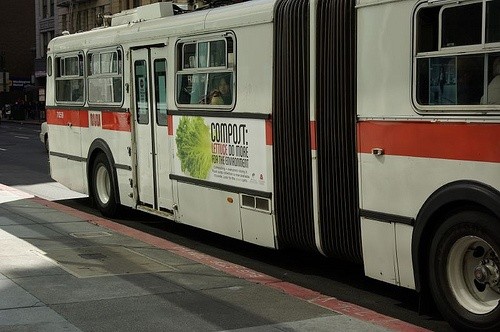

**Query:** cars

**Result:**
xmin=40 ymin=122 xmax=49 ymax=155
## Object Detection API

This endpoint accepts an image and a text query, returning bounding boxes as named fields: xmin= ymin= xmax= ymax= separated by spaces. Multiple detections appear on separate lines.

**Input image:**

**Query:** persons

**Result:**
xmin=209 ymin=76 xmax=232 ymax=105
xmin=2 ymin=98 xmax=40 ymax=121
xmin=480 ymin=57 xmax=500 ymax=105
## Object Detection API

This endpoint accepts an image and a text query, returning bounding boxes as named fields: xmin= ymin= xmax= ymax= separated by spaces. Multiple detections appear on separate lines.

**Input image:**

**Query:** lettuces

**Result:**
xmin=174 ymin=118 xmax=215 ymax=181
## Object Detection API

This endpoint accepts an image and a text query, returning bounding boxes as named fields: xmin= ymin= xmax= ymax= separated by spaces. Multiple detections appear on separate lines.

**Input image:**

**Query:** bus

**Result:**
xmin=46 ymin=1 xmax=500 ymax=332
